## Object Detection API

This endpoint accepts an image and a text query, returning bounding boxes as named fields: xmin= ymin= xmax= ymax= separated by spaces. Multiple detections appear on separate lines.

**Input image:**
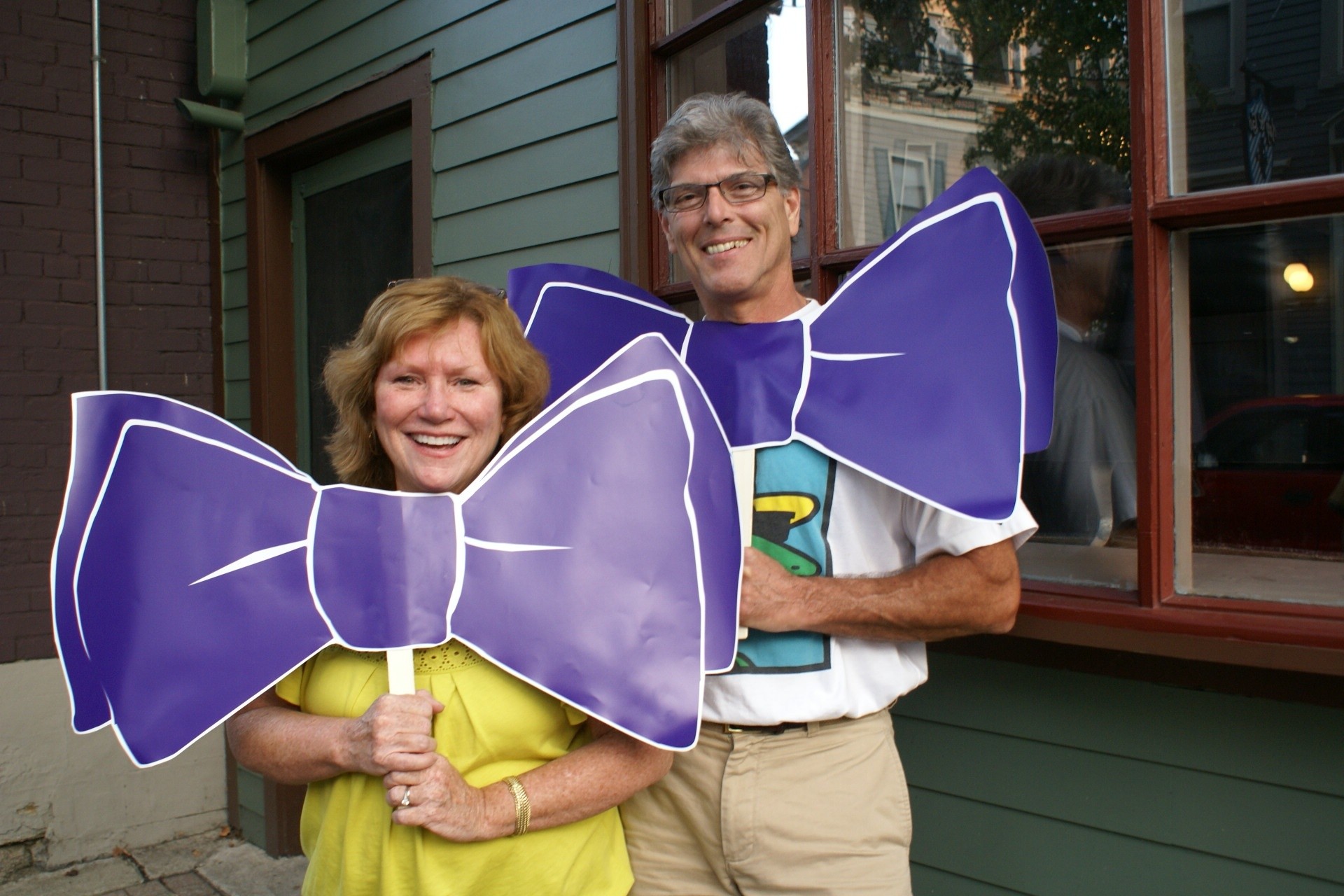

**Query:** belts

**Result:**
xmin=721 ymin=722 xmax=806 ymax=736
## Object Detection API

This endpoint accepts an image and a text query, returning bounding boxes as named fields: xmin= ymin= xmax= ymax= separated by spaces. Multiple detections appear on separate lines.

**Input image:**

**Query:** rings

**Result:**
xmin=400 ymin=785 xmax=411 ymax=806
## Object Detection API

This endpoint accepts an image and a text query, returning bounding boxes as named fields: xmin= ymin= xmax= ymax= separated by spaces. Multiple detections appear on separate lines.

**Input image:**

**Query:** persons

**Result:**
xmin=223 ymin=274 xmax=675 ymax=896
xmin=997 ymin=154 xmax=1142 ymax=533
xmin=591 ymin=92 xmax=1021 ymax=896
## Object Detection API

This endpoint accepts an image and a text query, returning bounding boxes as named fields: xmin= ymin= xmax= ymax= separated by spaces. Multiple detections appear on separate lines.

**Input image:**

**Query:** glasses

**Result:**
xmin=386 ymin=278 xmax=506 ymax=301
xmin=658 ymin=171 xmax=779 ymax=213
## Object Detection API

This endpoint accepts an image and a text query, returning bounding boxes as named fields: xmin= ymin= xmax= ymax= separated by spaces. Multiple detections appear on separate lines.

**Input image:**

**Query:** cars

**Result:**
xmin=1185 ymin=391 xmax=1343 ymax=559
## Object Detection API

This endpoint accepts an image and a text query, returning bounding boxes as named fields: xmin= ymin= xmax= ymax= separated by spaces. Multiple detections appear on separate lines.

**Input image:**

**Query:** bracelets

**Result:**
xmin=502 ymin=775 xmax=531 ymax=837
